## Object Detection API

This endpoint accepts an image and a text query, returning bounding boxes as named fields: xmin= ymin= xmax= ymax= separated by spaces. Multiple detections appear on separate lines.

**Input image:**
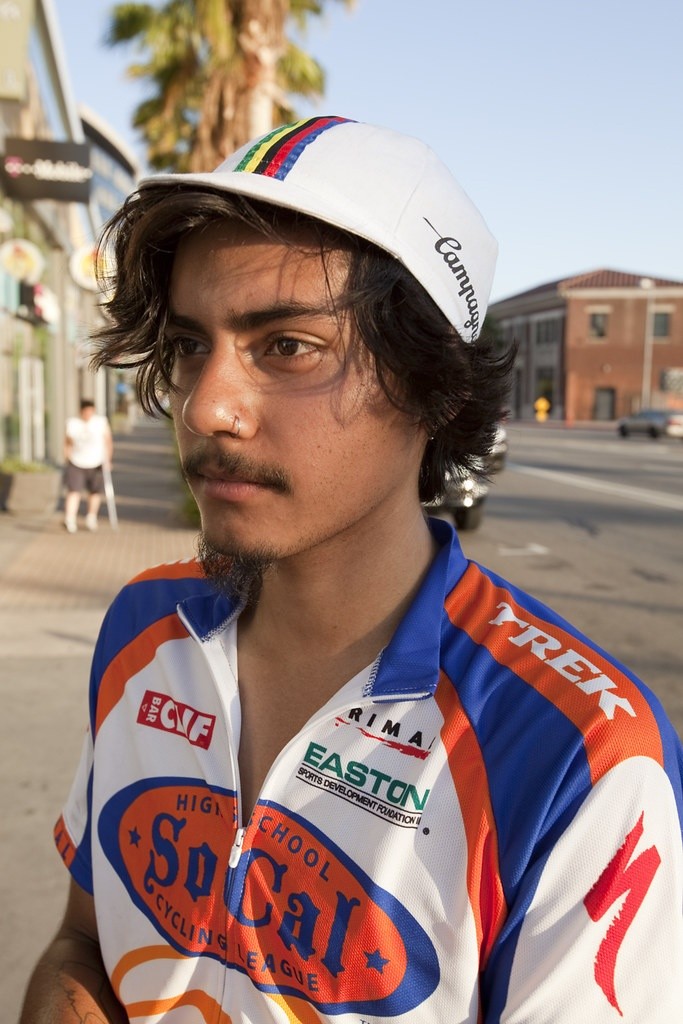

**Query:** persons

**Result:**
xmin=61 ymin=399 xmax=112 ymax=535
xmin=18 ymin=116 xmax=683 ymax=1024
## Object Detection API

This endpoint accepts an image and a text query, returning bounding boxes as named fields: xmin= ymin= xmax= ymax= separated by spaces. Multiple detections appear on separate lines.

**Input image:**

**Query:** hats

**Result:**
xmin=138 ymin=114 xmax=497 ymax=343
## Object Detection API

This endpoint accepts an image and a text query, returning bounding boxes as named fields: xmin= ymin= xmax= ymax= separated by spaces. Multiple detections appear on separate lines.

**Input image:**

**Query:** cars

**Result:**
xmin=618 ymin=410 xmax=683 ymax=439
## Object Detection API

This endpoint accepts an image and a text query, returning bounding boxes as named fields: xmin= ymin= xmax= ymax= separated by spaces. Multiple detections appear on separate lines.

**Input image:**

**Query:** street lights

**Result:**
xmin=640 ymin=277 xmax=656 ymax=413
